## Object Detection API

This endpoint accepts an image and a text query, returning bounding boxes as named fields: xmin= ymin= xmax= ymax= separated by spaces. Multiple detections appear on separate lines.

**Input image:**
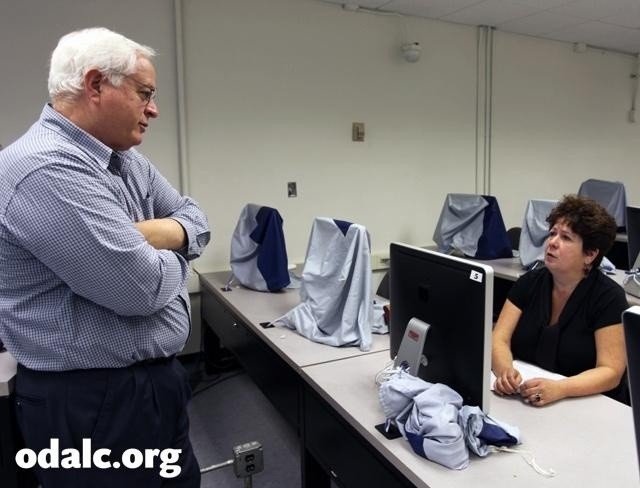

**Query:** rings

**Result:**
xmin=536 ymin=394 xmax=541 ymax=401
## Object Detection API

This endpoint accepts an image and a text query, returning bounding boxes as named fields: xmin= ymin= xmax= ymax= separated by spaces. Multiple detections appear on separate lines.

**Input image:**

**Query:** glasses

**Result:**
xmin=110 ymin=67 xmax=159 ymax=101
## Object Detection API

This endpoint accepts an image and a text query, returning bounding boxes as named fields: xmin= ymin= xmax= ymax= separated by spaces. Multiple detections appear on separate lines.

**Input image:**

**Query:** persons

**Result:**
xmin=0 ymin=27 xmax=211 ymax=488
xmin=491 ymin=194 xmax=627 ymax=407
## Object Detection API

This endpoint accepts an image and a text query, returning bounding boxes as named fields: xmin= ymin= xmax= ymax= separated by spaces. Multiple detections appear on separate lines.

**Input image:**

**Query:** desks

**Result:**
xmin=196 ymin=243 xmax=639 ymax=487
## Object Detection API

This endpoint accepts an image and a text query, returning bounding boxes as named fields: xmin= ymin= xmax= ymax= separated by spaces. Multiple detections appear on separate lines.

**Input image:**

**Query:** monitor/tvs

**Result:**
xmin=622 ymin=306 xmax=640 ymax=483
xmin=387 ymin=241 xmax=495 ymax=423
